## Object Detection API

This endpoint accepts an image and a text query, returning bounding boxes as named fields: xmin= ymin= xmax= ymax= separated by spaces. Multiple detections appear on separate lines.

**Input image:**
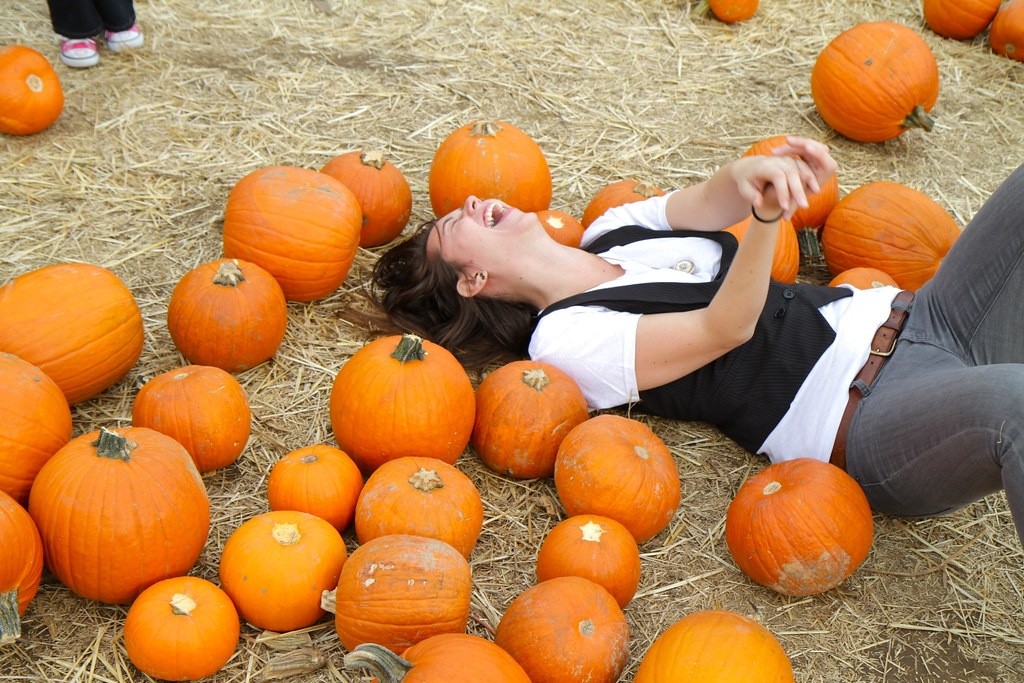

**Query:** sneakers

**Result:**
xmin=104 ymin=21 xmax=143 ymax=52
xmin=59 ymin=37 xmax=100 ymax=67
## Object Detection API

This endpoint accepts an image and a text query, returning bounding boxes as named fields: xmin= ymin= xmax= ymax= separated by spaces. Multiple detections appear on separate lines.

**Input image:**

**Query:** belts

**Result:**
xmin=830 ymin=290 xmax=916 ymax=472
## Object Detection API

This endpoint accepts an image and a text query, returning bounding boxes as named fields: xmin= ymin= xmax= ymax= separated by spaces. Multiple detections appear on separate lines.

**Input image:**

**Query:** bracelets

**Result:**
xmin=752 ymin=205 xmax=784 ymax=223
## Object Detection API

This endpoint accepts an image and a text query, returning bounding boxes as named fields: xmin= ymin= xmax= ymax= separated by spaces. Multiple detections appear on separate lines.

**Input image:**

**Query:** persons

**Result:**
xmin=373 ymin=136 xmax=1023 ymax=549
xmin=47 ymin=0 xmax=143 ymax=67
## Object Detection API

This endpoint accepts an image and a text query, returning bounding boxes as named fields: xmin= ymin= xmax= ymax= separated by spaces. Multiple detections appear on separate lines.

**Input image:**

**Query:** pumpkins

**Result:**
xmin=0 ymin=122 xmax=969 ymax=683
xmin=811 ymin=22 xmax=940 ymax=144
xmin=924 ymin=0 xmax=1024 ymax=62
xmin=707 ymin=0 xmax=759 ymax=23
xmin=1 ymin=44 xmax=64 ymax=136
